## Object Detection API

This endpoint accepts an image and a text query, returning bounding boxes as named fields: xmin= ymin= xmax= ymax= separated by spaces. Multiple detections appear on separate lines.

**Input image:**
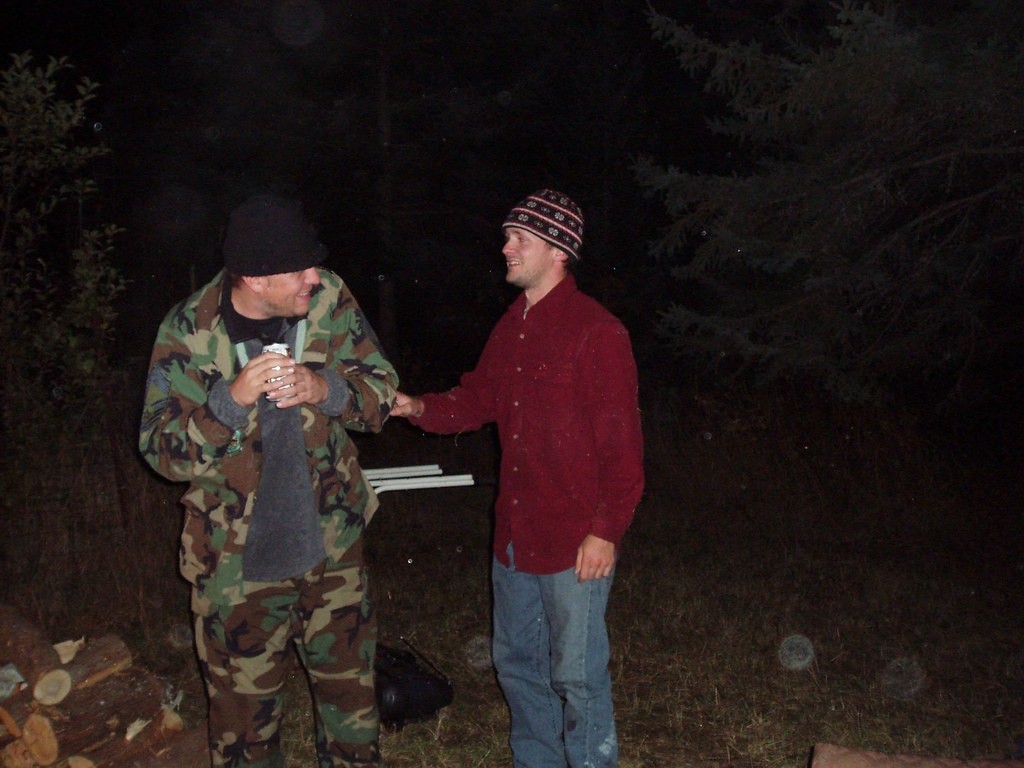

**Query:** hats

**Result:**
xmin=502 ymin=189 xmax=585 ymax=264
xmin=222 ymin=195 xmax=321 ymax=276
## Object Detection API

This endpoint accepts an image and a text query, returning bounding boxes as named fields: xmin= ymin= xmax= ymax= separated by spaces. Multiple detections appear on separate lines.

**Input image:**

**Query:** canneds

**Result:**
xmin=263 ymin=342 xmax=295 ymax=404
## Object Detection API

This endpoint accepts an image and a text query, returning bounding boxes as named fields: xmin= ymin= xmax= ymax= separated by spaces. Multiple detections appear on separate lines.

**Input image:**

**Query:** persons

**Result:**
xmin=138 ymin=198 xmax=400 ymax=768
xmin=387 ymin=189 xmax=645 ymax=768
